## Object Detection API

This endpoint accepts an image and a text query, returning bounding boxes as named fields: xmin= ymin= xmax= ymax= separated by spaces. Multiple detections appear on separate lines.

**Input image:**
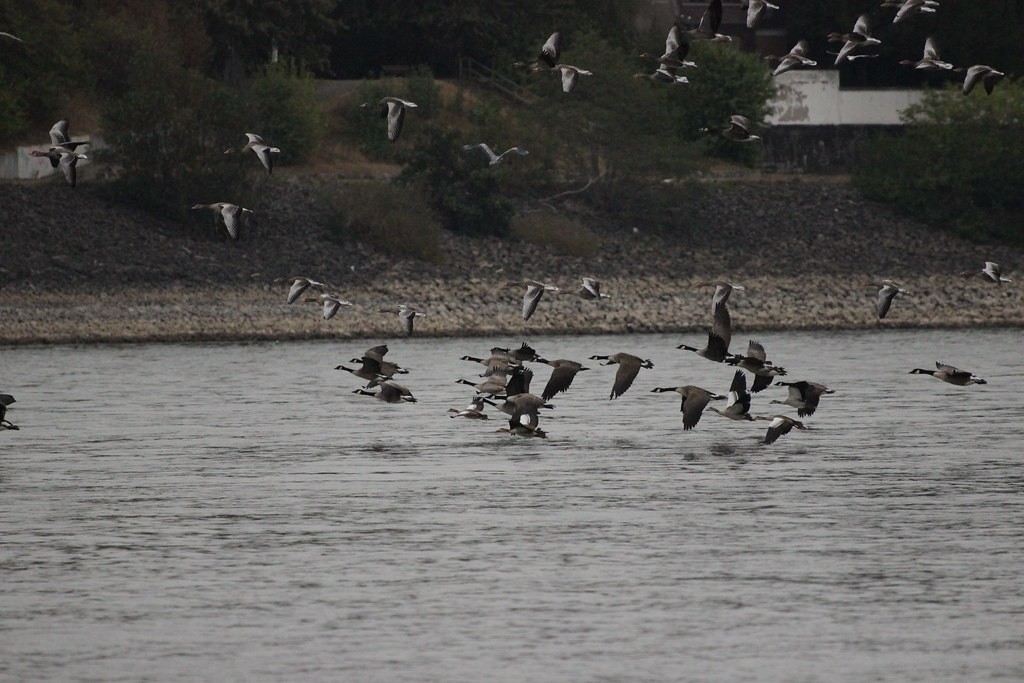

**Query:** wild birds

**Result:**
xmin=465 ymin=142 xmax=528 ymax=167
xmin=334 ymin=344 xmax=418 ymax=405
xmin=982 ymin=261 xmax=1013 ymax=284
xmin=447 ymin=342 xmax=590 ymax=440
xmin=557 ymin=277 xmax=612 ymax=302
xmin=300 ymin=293 xmax=354 ymax=321
xmin=1 ymin=30 xmax=26 ymax=45
xmin=699 ymin=114 xmax=762 ymax=143
xmin=878 ymin=279 xmax=910 ymax=319
xmin=504 ymin=277 xmax=559 ymax=321
xmin=633 ymin=0 xmax=1006 ymax=98
xmin=186 ymin=201 xmax=255 ymax=243
xmin=907 ymin=361 xmax=987 ymax=387
xmin=587 ymin=352 xmax=655 ymax=402
xmin=0 ymin=391 xmax=19 ymax=432
xmin=273 ymin=276 xmax=325 ymax=305
xmin=28 ymin=120 xmax=90 ymax=188
xmin=377 ymin=305 xmax=427 ymax=337
xmin=224 ymin=133 xmax=281 ymax=174
xmin=651 ymin=282 xmax=836 ymax=445
xmin=511 ymin=32 xmax=594 ymax=94
xmin=359 ymin=96 xmax=418 ymax=142
